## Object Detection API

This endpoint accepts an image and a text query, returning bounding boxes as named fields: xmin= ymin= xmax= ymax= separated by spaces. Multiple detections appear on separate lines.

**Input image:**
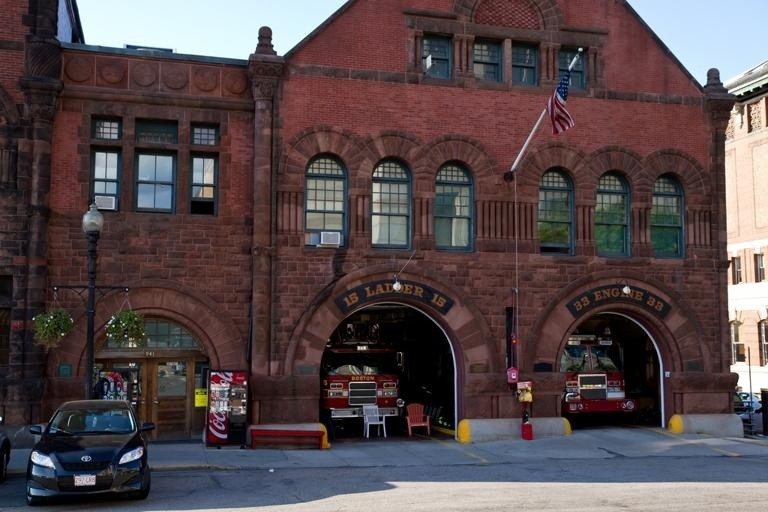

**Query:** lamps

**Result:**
xmin=391 ymin=248 xmax=416 ymax=291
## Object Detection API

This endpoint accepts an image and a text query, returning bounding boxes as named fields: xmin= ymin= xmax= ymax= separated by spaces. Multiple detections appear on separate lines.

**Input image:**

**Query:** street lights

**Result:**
xmin=82 ymin=204 xmax=103 ymax=400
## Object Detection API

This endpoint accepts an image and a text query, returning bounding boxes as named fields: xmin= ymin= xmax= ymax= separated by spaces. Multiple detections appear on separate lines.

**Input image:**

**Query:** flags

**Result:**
xmin=543 ymin=67 xmax=577 ymax=136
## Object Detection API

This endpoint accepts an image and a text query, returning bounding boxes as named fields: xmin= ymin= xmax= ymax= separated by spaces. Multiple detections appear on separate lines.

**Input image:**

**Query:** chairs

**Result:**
xmin=56 ymin=416 xmax=86 ymax=428
xmin=363 ymin=404 xmax=387 ymax=439
xmin=405 ymin=403 xmax=431 ymax=438
xmin=106 ymin=413 xmax=128 ymax=429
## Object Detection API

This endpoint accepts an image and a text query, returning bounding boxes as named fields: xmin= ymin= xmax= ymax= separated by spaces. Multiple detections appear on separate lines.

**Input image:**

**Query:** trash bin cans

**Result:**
xmin=758 ymin=388 xmax=768 ymax=436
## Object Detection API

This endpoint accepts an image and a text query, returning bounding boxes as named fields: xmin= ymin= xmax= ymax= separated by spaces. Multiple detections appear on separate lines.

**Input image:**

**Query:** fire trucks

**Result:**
xmin=560 ymin=318 xmax=637 ymax=413
xmin=319 ymin=309 xmax=407 ymax=430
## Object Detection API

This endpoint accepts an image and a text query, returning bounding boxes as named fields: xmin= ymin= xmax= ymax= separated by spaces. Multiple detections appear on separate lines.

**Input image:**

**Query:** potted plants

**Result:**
xmin=107 ymin=310 xmax=148 ymax=350
xmin=33 ymin=309 xmax=74 ymax=347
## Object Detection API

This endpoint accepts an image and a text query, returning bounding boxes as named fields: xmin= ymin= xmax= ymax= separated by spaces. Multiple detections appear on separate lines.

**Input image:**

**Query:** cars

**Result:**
xmin=0 ymin=416 xmax=10 ymax=480
xmin=734 ymin=392 xmax=764 ymax=439
xmin=27 ymin=400 xmax=155 ymax=504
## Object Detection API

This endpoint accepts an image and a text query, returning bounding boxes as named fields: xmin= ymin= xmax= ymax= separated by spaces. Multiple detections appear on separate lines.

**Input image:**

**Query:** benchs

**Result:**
xmin=250 ymin=423 xmax=323 ymax=450
